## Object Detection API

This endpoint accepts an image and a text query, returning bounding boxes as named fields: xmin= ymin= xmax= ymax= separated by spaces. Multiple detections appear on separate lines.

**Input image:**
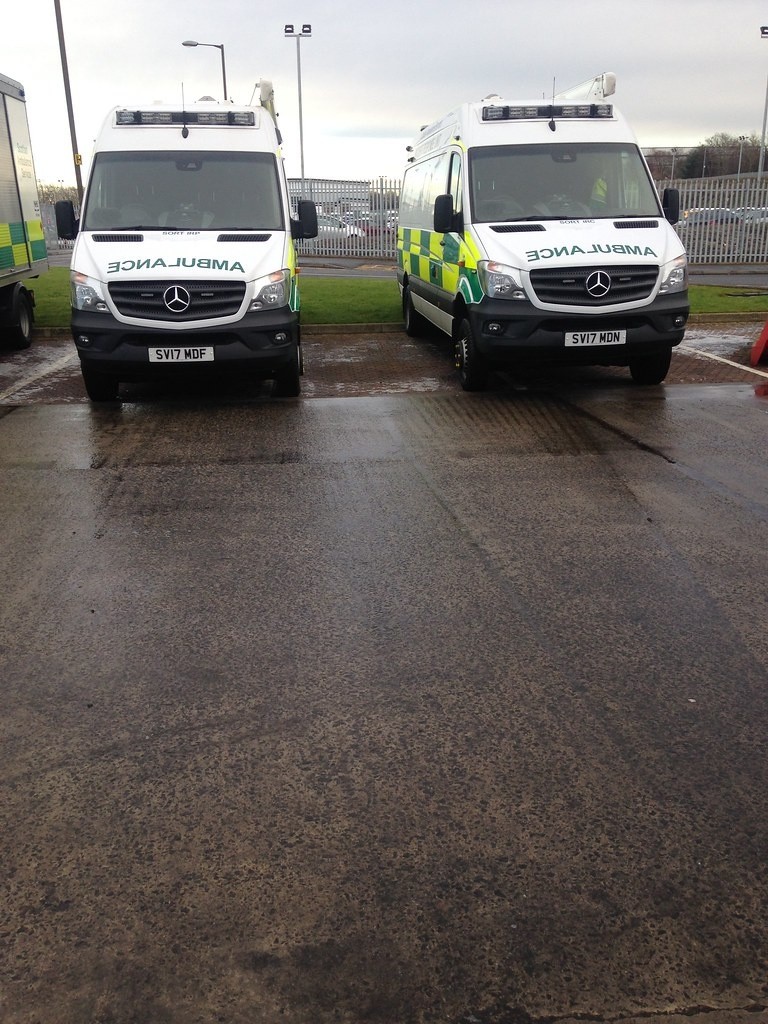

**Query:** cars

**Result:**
xmin=347 ymin=218 xmax=394 ymax=235
xmin=733 ymin=207 xmax=768 ymax=224
xmin=687 ymin=209 xmax=743 ymax=226
xmin=294 ymin=213 xmax=367 ymax=242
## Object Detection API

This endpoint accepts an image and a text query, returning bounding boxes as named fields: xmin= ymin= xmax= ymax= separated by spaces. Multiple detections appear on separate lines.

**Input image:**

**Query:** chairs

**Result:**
xmin=197 ymin=164 xmax=261 ymax=228
xmin=113 ymin=166 xmax=162 ymax=227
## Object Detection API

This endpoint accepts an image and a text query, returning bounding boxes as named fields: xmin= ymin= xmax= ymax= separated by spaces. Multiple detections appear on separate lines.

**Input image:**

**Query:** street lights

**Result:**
xmin=182 ymin=41 xmax=227 ymax=100
xmin=752 ymin=26 xmax=768 ymax=224
xmin=285 ymin=24 xmax=312 ymax=198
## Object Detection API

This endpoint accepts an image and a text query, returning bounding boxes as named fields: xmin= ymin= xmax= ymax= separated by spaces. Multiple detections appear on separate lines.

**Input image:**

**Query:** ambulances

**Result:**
xmin=396 ymin=72 xmax=691 ymax=392
xmin=0 ymin=73 xmax=49 ymax=353
xmin=69 ymin=76 xmax=320 ymax=401
